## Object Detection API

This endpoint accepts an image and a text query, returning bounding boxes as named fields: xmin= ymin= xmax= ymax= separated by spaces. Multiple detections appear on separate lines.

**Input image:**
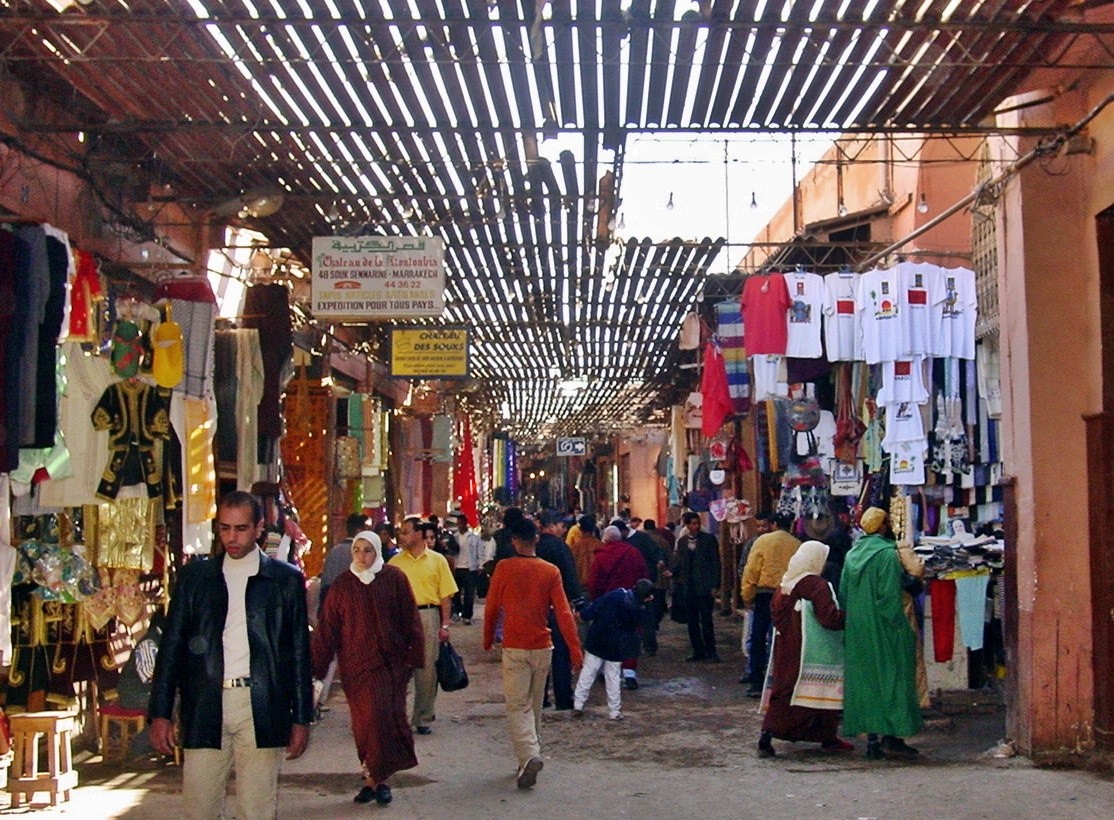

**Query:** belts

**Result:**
xmin=222 ymin=677 xmax=252 ymax=688
xmin=419 ymin=604 xmax=440 ymax=610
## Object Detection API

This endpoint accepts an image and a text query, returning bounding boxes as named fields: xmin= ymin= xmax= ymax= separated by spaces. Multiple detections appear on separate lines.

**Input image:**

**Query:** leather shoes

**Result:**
xmin=354 ymin=783 xmax=393 ymax=804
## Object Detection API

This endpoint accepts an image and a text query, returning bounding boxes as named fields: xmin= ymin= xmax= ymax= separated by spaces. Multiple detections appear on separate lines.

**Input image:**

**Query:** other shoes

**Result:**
xmin=739 ymin=672 xmax=764 ymax=684
xmin=608 ymin=710 xmax=624 ymax=721
xmin=572 ymin=710 xmax=584 ymax=719
xmin=315 ymin=706 xmax=331 ymax=720
xmin=418 ymin=726 xmax=433 ymax=735
xmin=544 ymin=699 xmax=575 ymax=711
xmin=598 ymin=671 xmax=637 ymax=688
xmin=686 ymin=652 xmax=718 ymax=662
xmin=757 ymin=740 xmax=776 ymax=756
xmin=820 ymin=736 xmax=855 ymax=753
xmin=453 ymin=613 xmax=472 ymax=625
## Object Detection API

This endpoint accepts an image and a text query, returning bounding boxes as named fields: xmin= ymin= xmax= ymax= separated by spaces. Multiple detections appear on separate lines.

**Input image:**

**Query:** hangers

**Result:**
xmin=759 ymin=250 xmax=956 ymax=275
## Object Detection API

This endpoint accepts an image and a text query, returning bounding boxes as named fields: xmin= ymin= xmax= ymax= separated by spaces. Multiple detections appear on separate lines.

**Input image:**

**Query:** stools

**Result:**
xmin=97 ymin=705 xmax=148 ymax=768
xmin=6 ymin=710 xmax=77 ymax=808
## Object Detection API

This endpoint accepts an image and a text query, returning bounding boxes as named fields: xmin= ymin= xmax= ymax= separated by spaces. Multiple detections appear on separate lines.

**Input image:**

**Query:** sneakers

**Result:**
xmin=516 ymin=757 xmax=543 ymax=788
xmin=865 ymin=734 xmax=918 ymax=760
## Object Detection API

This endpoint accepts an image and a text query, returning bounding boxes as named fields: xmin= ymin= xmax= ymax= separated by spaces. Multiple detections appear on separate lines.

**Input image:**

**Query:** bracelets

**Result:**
xmin=441 ymin=624 xmax=451 ymax=631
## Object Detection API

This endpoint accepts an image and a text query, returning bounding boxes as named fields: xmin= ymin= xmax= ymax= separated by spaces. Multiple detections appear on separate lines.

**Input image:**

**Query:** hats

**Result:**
xmin=859 ymin=506 xmax=886 ymax=534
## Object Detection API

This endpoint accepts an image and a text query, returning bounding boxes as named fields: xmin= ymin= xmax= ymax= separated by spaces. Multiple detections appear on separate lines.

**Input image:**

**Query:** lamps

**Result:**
xmin=214 ymin=180 xmax=287 ymax=219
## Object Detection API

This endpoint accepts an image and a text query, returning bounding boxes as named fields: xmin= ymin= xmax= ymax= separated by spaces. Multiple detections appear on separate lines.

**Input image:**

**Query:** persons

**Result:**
xmin=145 ymin=490 xmax=315 ymax=820
xmin=661 ymin=512 xmax=722 ymax=664
xmin=312 ymin=537 xmax=354 ymax=721
xmin=116 ymin=616 xmax=177 ymax=770
xmin=737 ymin=505 xmax=931 ymax=758
xmin=374 ymin=503 xmax=722 ymax=735
xmin=482 ymin=518 xmax=584 ymax=789
xmin=758 ymin=539 xmax=856 ymax=755
xmin=385 ymin=517 xmax=460 ymax=735
xmin=310 ymin=530 xmax=426 ymax=805
xmin=570 ymin=578 xmax=658 ymax=721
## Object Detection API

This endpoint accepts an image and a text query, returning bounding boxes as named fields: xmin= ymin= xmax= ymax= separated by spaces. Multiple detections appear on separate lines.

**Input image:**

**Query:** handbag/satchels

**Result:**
xmin=685 ymin=461 xmax=716 ymax=512
xmin=435 ymin=640 xmax=469 ymax=692
xmin=670 ymin=588 xmax=689 ymax=624
xmin=776 ymin=431 xmax=833 ymax=518
